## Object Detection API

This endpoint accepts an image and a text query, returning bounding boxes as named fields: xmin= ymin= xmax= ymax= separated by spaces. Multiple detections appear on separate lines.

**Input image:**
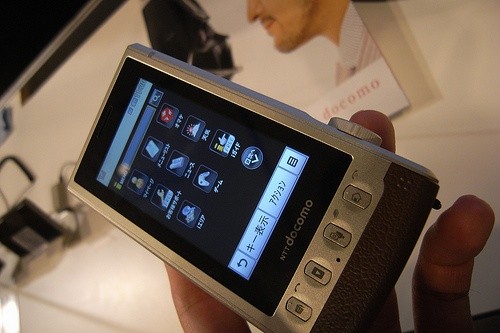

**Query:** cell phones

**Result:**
xmin=66 ymin=42 xmax=441 ymax=332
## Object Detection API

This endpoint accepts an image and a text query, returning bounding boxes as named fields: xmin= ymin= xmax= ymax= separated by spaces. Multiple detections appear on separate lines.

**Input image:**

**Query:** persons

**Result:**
xmin=246 ymin=1 xmax=383 ymax=89
xmin=162 ymin=110 xmax=500 ymax=333
